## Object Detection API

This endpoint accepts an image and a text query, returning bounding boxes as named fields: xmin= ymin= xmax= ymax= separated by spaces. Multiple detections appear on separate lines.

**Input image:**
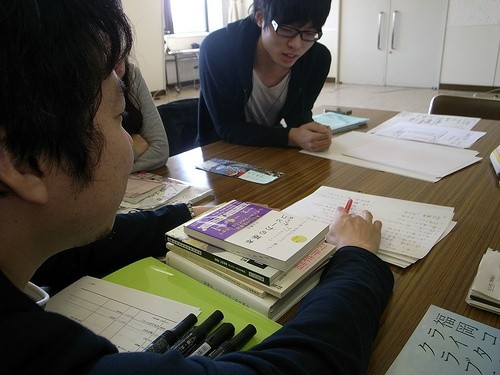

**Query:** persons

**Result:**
xmin=0 ymin=0 xmax=394 ymax=375
xmin=199 ymin=0 xmax=332 ymax=152
xmin=112 ymin=44 xmax=170 ymax=173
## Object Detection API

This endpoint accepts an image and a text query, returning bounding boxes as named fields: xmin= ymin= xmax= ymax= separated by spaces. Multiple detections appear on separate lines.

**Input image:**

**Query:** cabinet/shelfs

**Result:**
xmin=313 ymin=0 xmax=500 ymax=90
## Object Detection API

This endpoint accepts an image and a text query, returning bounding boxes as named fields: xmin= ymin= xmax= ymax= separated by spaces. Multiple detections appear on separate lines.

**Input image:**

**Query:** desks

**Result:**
xmin=164 ymin=48 xmax=201 ymax=94
xmin=116 ymin=104 xmax=500 ymax=375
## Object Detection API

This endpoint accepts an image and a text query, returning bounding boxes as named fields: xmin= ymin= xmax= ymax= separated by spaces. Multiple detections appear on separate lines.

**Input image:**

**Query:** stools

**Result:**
xmin=192 ymin=64 xmax=199 ymax=88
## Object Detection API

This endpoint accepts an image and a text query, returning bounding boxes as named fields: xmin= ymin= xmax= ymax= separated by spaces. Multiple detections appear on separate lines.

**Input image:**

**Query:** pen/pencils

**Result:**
xmin=325 ymin=199 xmax=352 ymax=242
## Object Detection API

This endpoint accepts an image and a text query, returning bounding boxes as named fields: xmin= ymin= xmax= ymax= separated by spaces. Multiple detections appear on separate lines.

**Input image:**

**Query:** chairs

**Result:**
xmin=428 ymin=94 xmax=500 ymax=121
xmin=155 ymin=97 xmax=201 ymax=158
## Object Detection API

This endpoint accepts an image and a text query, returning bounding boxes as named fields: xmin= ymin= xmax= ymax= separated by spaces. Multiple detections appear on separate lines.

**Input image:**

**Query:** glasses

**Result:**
xmin=267 ymin=12 xmax=324 ymax=41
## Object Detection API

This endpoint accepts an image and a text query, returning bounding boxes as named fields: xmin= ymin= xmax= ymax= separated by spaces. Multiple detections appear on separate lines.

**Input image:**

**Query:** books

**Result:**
xmin=98 ymin=199 xmax=342 ymax=352
xmin=385 ymin=246 xmax=500 ymax=375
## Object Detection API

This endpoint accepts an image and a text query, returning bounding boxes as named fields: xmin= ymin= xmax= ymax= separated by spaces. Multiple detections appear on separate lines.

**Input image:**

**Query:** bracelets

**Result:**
xmin=186 ymin=203 xmax=196 ymax=218
